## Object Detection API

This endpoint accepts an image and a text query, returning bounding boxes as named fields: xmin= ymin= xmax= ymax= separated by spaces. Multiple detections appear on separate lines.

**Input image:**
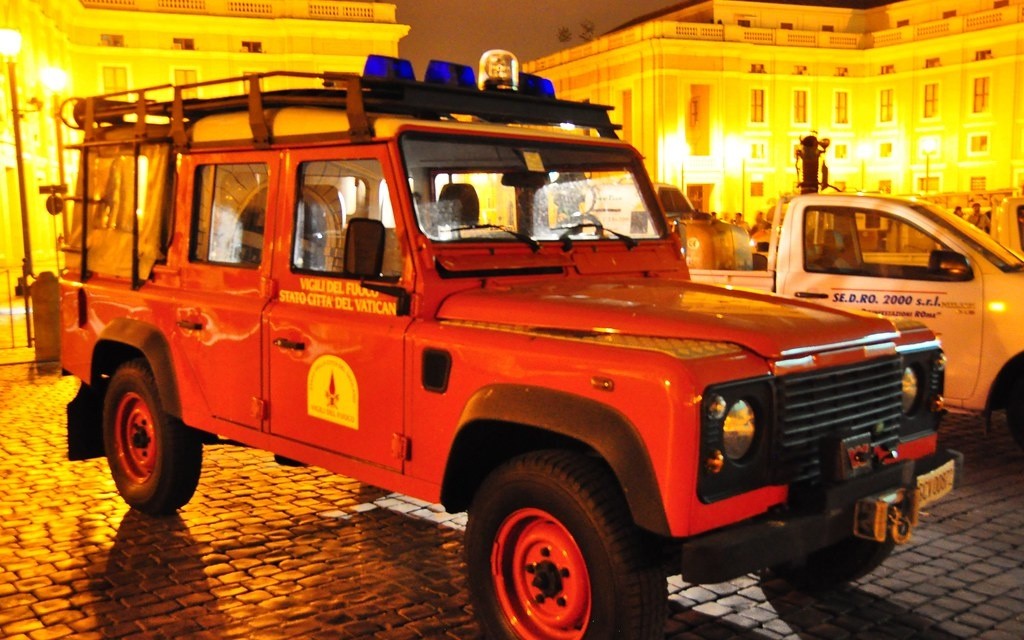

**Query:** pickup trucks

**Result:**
xmin=687 ymin=189 xmax=1024 ymax=450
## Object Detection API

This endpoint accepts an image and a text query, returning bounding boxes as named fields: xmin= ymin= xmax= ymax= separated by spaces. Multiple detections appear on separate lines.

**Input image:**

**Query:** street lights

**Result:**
xmin=920 ymin=138 xmax=940 ymax=193
xmin=0 ymin=31 xmax=33 ymax=285
xmin=42 ymin=68 xmax=69 ymax=243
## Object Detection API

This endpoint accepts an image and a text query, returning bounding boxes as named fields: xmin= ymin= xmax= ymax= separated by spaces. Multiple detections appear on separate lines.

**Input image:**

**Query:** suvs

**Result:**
xmin=46 ymin=49 xmax=964 ymax=640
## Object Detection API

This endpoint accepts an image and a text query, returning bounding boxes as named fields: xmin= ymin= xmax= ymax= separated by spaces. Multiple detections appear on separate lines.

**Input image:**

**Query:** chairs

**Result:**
xmin=804 ymin=223 xmax=821 ymax=271
xmin=438 ymin=183 xmax=480 ymax=227
xmin=823 ymin=229 xmax=857 ymax=270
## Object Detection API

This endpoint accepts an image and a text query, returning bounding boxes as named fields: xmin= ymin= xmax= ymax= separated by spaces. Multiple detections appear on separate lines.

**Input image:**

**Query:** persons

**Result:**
xmin=954 ymin=203 xmax=993 ymax=234
xmin=712 ymin=211 xmax=772 ymax=251
